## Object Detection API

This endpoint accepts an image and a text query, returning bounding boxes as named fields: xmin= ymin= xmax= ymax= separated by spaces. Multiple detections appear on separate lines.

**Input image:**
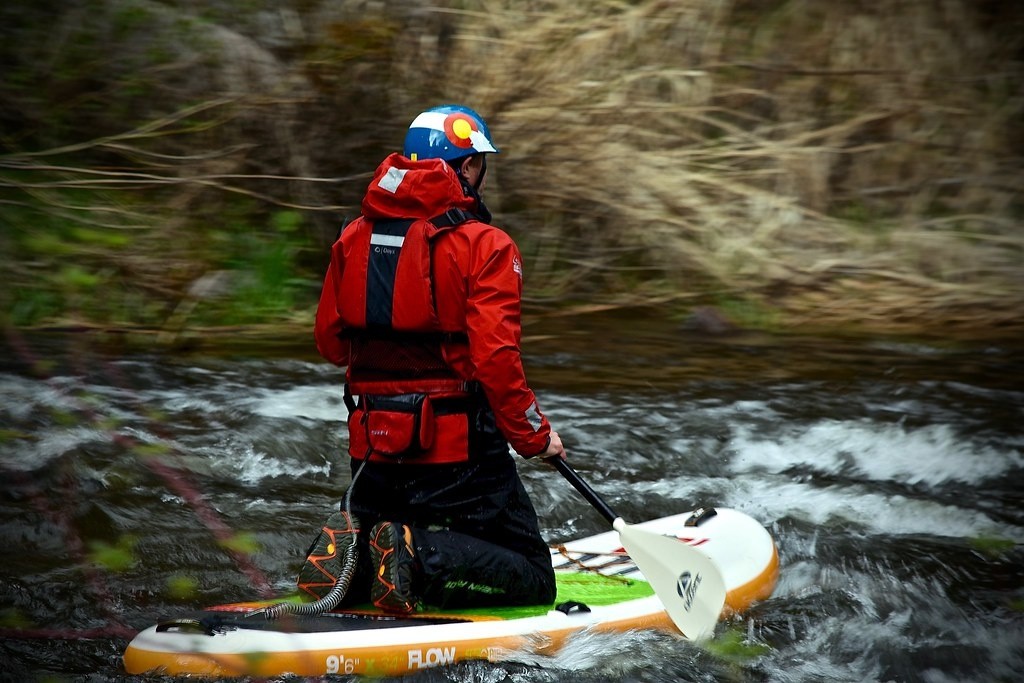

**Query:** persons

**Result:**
xmin=299 ymin=104 xmax=567 ymax=614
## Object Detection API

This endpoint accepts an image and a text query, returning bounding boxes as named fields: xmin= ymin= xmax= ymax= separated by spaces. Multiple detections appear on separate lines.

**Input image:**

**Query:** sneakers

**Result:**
xmin=369 ymin=521 xmax=418 ymax=615
xmin=297 ymin=511 xmax=360 ymax=604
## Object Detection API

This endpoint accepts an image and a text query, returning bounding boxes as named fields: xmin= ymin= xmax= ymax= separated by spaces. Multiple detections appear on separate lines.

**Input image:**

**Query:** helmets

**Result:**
xmin=403 ymin=104 xmax=503 ymax=162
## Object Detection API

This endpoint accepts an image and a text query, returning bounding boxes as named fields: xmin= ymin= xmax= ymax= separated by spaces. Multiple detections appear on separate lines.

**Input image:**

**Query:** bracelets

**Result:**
xmin=539 ymin=436 xmax=551 ymax=454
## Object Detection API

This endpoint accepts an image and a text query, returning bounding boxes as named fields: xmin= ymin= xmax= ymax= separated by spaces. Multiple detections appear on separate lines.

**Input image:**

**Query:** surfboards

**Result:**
xmin=121 ymin=504 xmax=783 ymax=680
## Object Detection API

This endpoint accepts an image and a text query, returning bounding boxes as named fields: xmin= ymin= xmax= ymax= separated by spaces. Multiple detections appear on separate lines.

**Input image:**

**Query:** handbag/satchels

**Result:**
xmin=363 ymin=389 xmax=433 ymax=457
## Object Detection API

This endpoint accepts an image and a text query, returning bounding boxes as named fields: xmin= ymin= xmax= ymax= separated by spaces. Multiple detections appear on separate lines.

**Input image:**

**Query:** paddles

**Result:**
xmin=547 ymin=455 xmax=730 ymax=643
